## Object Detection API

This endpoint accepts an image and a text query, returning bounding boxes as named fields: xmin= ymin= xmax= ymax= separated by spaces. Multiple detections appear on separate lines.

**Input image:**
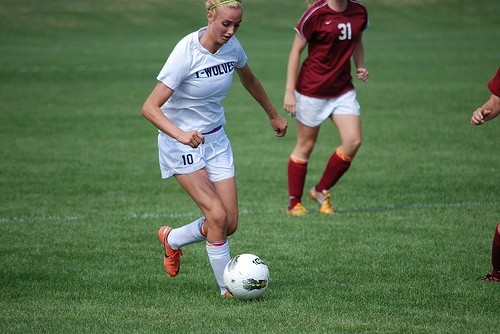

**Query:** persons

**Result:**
xmin=471 ymin=68 xmax=500 ymax=284
xmin=283 ymin=0 xmax=369 ymax=217
xmin=141 ymin=0 xmax=288 ymax=299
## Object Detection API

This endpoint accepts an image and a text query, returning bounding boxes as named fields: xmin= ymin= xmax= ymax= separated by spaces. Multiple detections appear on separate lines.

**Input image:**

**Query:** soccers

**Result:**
xmin=224 ymin=251 xmax=271 ymax=300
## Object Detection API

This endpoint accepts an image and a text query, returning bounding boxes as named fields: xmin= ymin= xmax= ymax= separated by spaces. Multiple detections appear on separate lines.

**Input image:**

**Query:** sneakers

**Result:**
xmin=288 ymin=202 xmax=309 ymax=217
xmin=308 ymin=185 xmax=333 ymax=215
xmin=157 ymin=225 xmax=183 ymax=278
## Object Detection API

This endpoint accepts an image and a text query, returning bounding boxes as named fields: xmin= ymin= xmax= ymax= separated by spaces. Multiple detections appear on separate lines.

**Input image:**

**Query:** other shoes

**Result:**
xmin=475 ymin=273 xmax=500 ymax=283
